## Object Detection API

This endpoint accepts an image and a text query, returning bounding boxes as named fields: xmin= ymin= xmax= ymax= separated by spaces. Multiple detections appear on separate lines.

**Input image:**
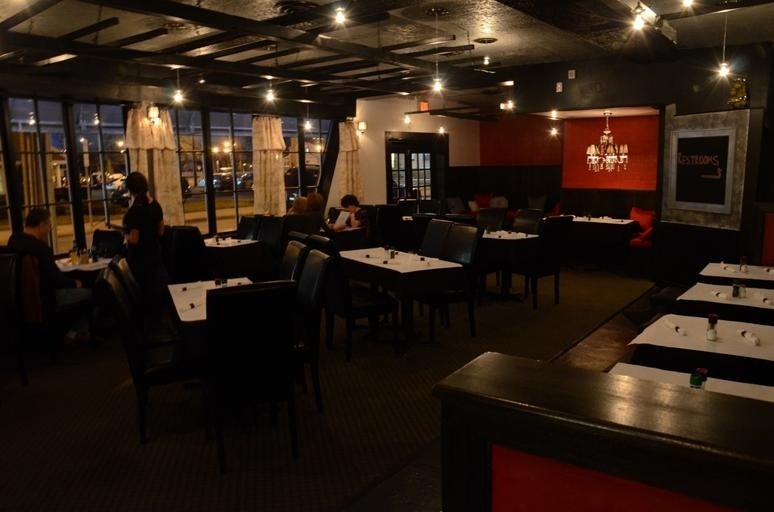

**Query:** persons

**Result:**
xmin=5 ymin=207 xmax=104 ymax=347
xmin=297 ymin=192 xmax=343 ymax=236
xmin=340 ymin=196 xmax=372 ymax=237
xmin=285 ymin=197 xmax=314 ymax=232
xmin=115 ymin=172 xmax=164 ymax=316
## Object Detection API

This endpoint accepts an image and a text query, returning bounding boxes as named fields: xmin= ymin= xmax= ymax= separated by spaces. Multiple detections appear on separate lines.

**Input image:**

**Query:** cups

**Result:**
xmin=69 ymin=248 xmax=89 ymax=266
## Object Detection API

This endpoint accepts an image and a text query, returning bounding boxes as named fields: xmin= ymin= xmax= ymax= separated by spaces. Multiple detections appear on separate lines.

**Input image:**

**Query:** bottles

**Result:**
xmin=71 ymin=239 xmax=76 ymax=251
xmin=212 ymin=279 xmax=228 ymax=289
xmin=732 ymin=282 xmax=747 ymax=298
xmin=382 ymin=246 xmax=395 ymax=261
xmin=706 ymin=315 xmax=718 ymax=341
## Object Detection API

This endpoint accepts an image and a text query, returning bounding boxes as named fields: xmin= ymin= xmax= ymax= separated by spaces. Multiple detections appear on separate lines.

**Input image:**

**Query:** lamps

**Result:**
xmin=587 ymin=113 xmax=630 ymax=173
xmin=633 ymin=1 xmax=646 ymax=33
xmin=264 ymin=80 xmax=276 ymax=104
xmin=173 ymin=68 xmax=184 ymax=103
xmin=305 ymin=89 xmax=312 ymax=131
xmin=358 ymin=121 xmax=368 ymax=136
xmin=432 ymin=15 xmax=443 ymax=93
xmin=717 ymin=14 xmax=731 ymax=80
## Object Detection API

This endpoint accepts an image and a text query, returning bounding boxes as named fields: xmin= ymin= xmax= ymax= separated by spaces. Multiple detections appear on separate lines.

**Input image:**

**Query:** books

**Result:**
xmin=333 ymin=211 xmax=350 ymax=226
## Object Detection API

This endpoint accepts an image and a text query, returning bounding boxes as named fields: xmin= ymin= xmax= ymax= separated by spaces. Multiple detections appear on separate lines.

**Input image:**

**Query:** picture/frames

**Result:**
xmin=667 ymin=126 xmax=736 ymax=216
